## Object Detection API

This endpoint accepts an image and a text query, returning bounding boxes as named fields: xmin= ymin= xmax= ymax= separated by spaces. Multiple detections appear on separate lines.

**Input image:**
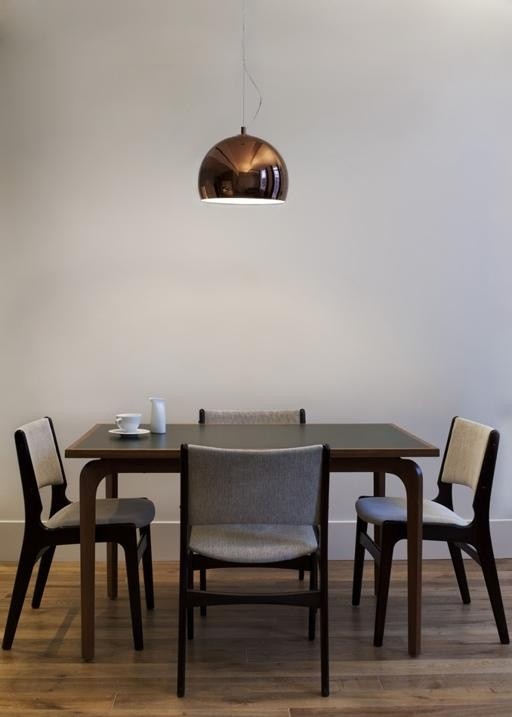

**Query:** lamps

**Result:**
xmin=198 ymin=1 xmax=288 ymax=206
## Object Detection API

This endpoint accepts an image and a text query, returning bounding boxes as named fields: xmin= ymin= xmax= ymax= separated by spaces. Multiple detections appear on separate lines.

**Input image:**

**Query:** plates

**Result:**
xmin=108 ymin=428 xmax=151 ymax=435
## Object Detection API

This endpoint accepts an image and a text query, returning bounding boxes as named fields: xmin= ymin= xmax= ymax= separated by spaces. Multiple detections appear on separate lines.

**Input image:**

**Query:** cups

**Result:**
xmin=149 ymin=397 xmax=166 ymax=434
xmin=114 ymin=413 xmax=142 ymax=432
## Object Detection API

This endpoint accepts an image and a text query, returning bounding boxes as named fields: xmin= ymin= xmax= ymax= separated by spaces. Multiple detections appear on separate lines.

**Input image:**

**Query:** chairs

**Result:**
xmin=352 ymin=417 xmax=509 ymax=649
xmin=177 ymin=443 xmax=329 ymax=696
xmin=3 ymin=415 xmax=155 ymax=650
xmin=199 ymin=408 xmax=304 ymax=617
